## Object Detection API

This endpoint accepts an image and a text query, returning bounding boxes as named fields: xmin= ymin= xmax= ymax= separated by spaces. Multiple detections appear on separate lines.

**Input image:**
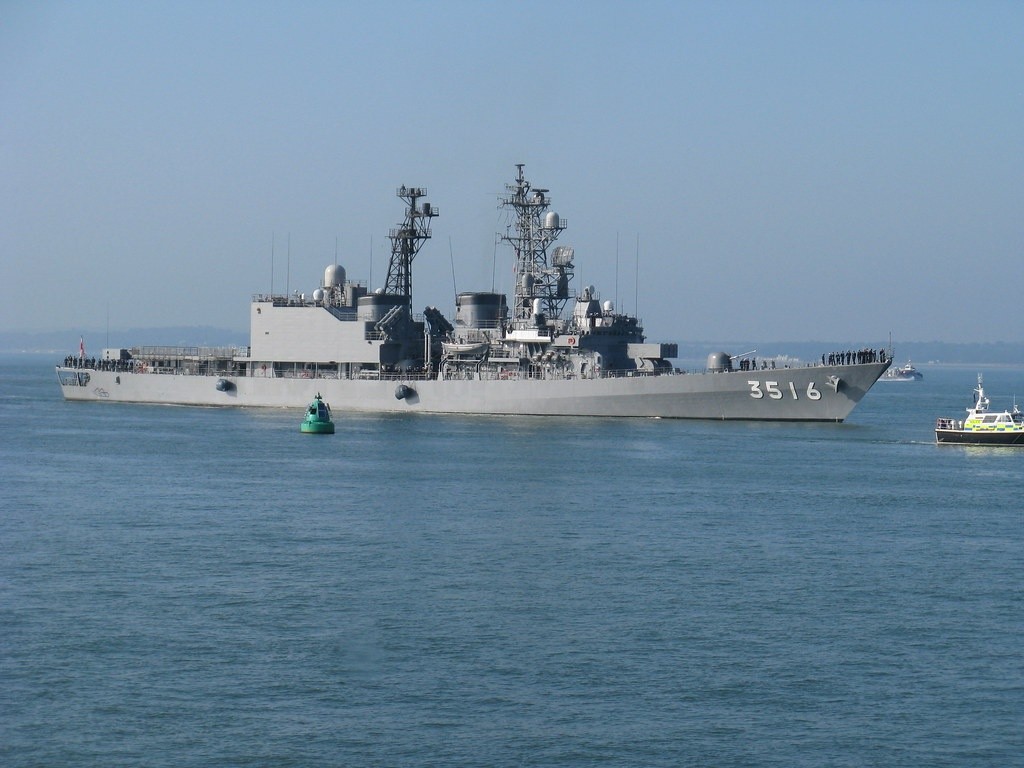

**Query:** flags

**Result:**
xmin=79 ymin=338 xmax=84 ymax=357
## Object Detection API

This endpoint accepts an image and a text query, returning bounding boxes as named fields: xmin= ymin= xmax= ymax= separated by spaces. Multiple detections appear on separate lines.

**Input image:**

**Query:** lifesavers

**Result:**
xmin=567 ymin=337 xmax=575 ymax=344
xmin=937 ymin=418 xmax=941 ymax=427
xmin=261 ymin=364 xmax=266 ymax=371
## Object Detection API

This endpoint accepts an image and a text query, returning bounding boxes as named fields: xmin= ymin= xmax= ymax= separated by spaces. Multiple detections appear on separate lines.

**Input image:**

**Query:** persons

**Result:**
xmin=822 ymin=348 xmax=887 ymax=366
xmin=63 ymin=355 xmax=134 ymax=373
xmin=740 ymin=357 xmax=775 ymax=372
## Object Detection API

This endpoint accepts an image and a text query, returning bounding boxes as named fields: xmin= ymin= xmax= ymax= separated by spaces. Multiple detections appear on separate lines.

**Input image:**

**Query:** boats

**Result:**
xmin=933 ymin=370 xmax=1024 ymax=448
xmin=55 ymin=162 xmax=897 ymax=424
xmin=440 ymin=338 xmax=492 ymax=356
xmin=883 ymin=353 xmax=924 ymax=382
